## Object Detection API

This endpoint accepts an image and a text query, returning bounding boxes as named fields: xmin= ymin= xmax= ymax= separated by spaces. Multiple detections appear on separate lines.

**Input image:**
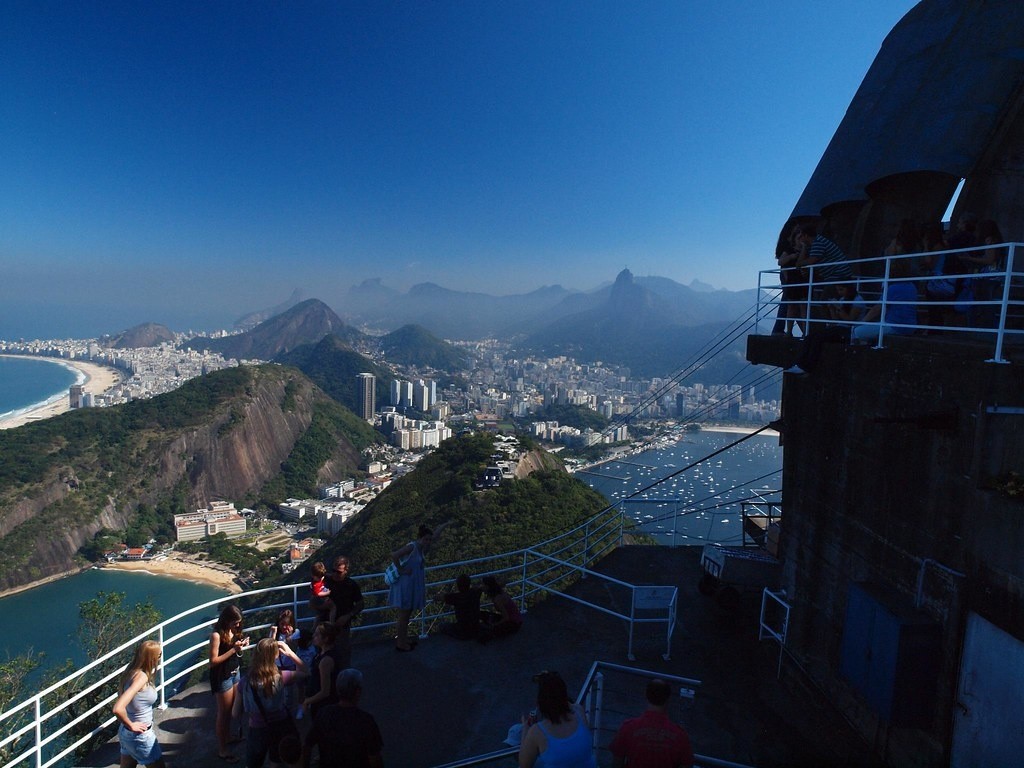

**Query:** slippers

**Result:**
xmin=217 ymin=755 xmax=239 ymax=764
xmin=227 ymin=735 xmax=241 ymax=744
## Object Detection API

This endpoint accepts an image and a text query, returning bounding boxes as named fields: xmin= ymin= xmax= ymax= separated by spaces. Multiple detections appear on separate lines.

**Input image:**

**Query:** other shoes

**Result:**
xmin=783 ymin=364 xmax=807 ymax=376
xmin=411 ymin=640 xmax=418 ymax=645
xmin=395 ymin=642 xmax=415 ymax=652
xmin=296 ymin=711 xmax=303 ymax=720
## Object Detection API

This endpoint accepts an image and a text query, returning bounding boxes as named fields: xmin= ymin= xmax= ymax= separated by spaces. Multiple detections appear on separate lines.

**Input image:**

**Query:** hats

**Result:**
xmin=502 ymin=723 xmax=522 ymax=746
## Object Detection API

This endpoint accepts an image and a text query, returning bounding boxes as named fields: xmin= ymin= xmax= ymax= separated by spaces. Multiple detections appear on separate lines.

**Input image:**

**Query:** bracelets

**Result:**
xmin=236 ymin=651 xmax=243 ymax=657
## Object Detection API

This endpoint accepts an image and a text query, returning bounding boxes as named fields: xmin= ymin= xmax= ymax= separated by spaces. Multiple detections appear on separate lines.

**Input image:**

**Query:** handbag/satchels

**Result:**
xmin=268 ymin=715 xmax=300 ymax=752
xmin=927 ymin=279 xmax=955 ymax=297
xmin=384 ymin=543 xmax=416 ymax=585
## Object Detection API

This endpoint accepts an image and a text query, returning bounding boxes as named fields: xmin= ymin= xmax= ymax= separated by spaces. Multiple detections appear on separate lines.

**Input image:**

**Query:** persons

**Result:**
xmin=210 ymin=605 xmax=249 ymax=763
xmin=112 ymin=640 xmax=166 ymax=768
xmin=608 ymin=678 xmax=693 ymax=768
xmin=386 ymin=525 xmax=433 ymax=653
xmin=231 ymin=609 xmax=385 ymax=768
xmin=502 ymin=723 xmax=525 ymax=768
xmin=308 ymin=559 xmax=365 ymax=669
xmin=437 ymin=574 xmax=522 ymax=641
xmin=519 ymin=675 xmax=589 ymax=768
xmin=771 ymin=218 xmax=1008 ymax=339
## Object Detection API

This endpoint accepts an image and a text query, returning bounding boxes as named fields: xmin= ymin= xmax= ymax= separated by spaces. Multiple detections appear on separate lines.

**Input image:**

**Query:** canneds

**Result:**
xmin=527 ymin=711 xmax=537 ymax=727
